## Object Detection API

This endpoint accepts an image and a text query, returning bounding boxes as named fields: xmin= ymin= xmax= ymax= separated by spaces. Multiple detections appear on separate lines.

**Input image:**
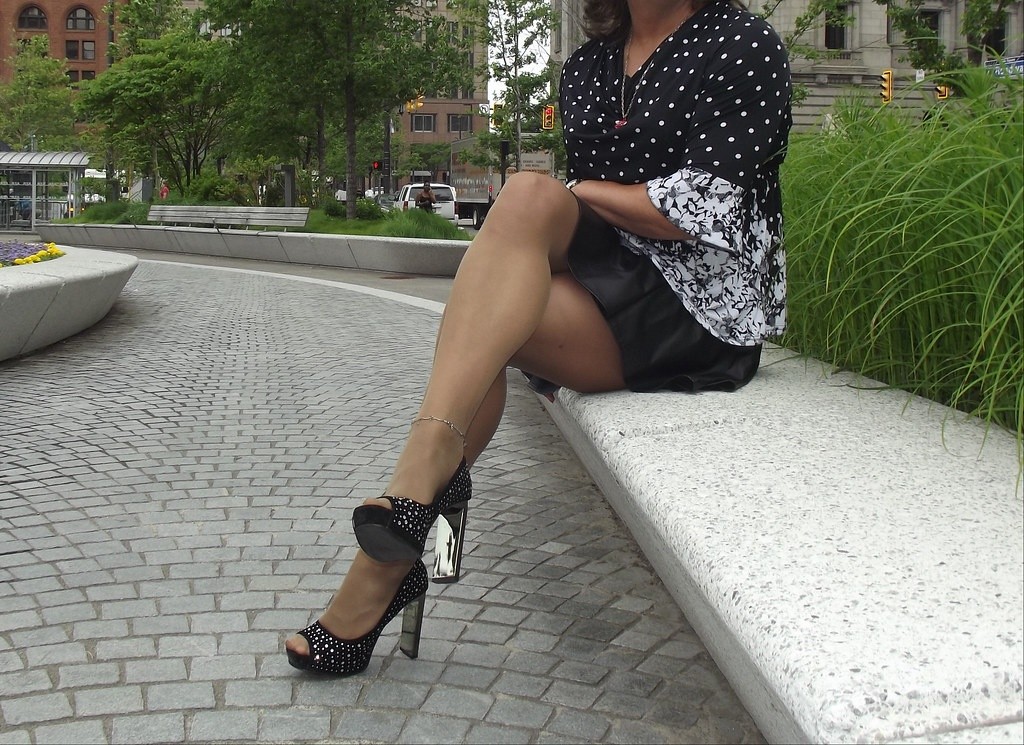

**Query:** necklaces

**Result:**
xmin=614 ymin=6 xmax=701 ymax=128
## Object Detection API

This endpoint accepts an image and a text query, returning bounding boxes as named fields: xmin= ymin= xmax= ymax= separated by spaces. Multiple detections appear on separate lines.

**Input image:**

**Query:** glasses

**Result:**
xmin=424 ymin=185 xmax=430 ymax=187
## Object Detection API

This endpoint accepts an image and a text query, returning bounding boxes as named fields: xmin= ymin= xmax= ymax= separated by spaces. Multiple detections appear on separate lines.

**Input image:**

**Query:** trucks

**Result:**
xmin=446 ymin=132 xmax=556 ymax=230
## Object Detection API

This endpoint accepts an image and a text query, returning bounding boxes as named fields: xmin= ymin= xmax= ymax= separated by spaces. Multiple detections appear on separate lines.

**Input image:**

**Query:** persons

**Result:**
xmin=413 ymin=179 xmax=437 ymax=217
xmin=158 ymin=181 xmax=172 ymax=200
xmin=282 ymin=0 xmax=795 ymax=677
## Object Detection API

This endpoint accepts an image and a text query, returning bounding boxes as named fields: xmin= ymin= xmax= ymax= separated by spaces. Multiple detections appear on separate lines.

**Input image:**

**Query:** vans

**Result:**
xmin=394 ymin=182 xmax=460 ymax=230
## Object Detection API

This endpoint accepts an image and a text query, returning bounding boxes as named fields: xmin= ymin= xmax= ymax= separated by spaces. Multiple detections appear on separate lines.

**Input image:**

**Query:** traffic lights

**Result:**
xmin=488 ymin=185 xmax=493 ymax=192
xmin=405 ymin=88 xmax=425 ymax=114
xmin=543 ymin=105 xmax=553 ymax=129
xmin=373 ymin=161 xmax=384 ymax=171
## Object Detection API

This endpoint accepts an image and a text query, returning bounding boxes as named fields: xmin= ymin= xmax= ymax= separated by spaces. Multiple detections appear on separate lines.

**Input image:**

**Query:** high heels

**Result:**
xmin=352 ymin=457 xmax=472 ymax=584
xmin=286 ymin=556 xmax=429 ymax=678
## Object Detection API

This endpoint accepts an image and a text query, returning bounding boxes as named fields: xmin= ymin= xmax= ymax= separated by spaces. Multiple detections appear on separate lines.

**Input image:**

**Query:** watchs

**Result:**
xmin=565 ymin=177 xmax=585 ymax=191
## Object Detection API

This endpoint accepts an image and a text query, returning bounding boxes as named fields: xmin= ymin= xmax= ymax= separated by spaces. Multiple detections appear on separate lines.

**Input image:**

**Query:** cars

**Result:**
xmin=365 ymin=186 xmax=385 ymax=198
xmin=374 ymin=194 xmax=397 ymax=211
